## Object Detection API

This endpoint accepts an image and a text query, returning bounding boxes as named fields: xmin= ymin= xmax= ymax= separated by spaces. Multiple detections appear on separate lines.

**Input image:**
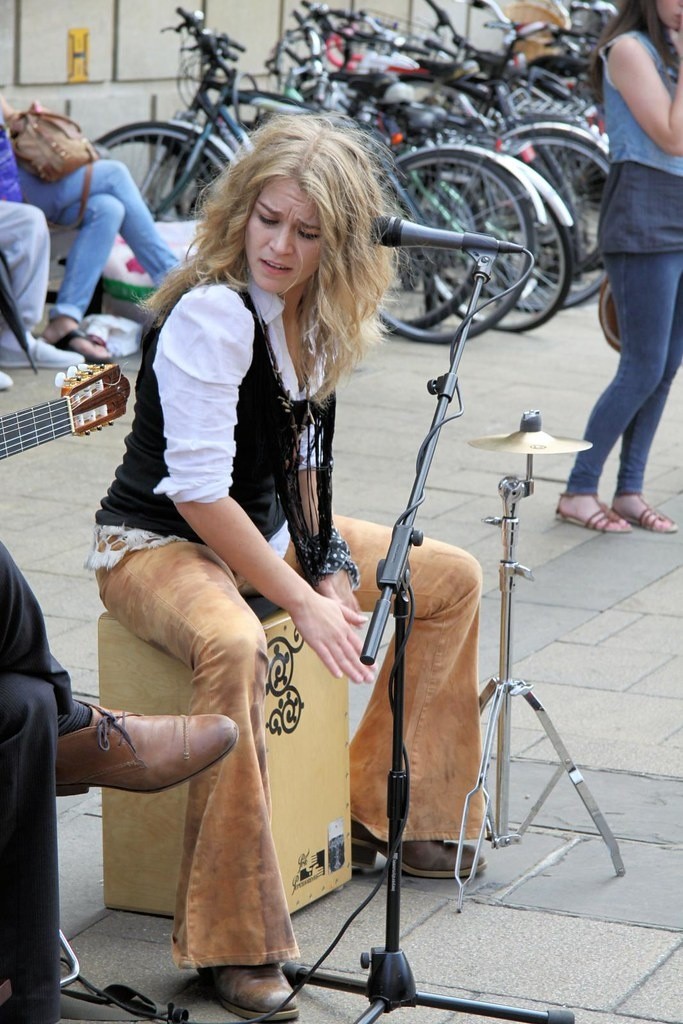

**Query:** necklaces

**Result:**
xmin=244 ymin=268 xmax=335 ymax=583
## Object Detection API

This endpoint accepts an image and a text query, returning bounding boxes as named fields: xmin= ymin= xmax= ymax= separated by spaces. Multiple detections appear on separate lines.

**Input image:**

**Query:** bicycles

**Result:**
xmin=92 ymin=1 xmax=623 ymax=347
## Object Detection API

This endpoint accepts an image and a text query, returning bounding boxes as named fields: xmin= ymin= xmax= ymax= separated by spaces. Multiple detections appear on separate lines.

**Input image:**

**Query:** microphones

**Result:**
xmin=369 ymin=215 xmax=524 ymax=253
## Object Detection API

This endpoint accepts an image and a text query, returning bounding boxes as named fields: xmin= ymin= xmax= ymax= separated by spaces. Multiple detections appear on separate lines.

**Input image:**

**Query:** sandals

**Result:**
xmin=553 ymin=492 xmax=633 ymax=534
xmin=611 ymin=493 xmax=678 ymax=532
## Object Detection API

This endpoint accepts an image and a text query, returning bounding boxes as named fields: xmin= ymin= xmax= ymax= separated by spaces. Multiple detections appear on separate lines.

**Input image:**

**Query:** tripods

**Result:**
xmin=479 ymin=453 xmax=627 ymax=876
xmin=282 ymin=252 xmax=577 ymax=1024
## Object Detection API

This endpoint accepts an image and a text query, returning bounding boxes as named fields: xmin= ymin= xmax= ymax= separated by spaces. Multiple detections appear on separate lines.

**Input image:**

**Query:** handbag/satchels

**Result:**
xmin=6 ymin=104 xmax=100 ymax=231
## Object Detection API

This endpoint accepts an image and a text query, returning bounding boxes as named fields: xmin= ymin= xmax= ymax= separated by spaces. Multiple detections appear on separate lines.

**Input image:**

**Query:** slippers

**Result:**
xmin=44 ymin=329 xmax=115 ymax=363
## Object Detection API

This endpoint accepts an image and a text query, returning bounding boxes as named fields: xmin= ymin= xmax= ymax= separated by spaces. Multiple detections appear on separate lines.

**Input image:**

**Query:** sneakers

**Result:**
xmin=1 ymin=371 xmax=13 ymax=390
xmin=1 ymin=339 xmax=85 ymax=368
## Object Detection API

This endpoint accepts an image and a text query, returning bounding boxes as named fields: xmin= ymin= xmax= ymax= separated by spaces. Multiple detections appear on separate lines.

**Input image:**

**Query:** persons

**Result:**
xmin=0 ymin=538 xmax=238 ymax=1023
xmin=83 ymin=114 xmax=488 ymax=1021
xmin=552 ymin=0 xmax=683 ymax=539
xmin=0 ymin=89 xmax=196 ymax=391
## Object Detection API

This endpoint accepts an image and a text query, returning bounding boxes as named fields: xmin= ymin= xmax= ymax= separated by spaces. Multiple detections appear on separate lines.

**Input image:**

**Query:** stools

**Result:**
xmin=95 ymin=598 xmax=353 ymax=918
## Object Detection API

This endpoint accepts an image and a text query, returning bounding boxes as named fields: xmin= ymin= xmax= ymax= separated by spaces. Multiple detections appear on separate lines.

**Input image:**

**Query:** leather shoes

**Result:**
xmin=196 ymin=952 xmax=299 ymax=1021
xmin=56 ymin=699 xmax=240 ymax=796
xmin=351 ymin=817 xmax=486 ymax=878
